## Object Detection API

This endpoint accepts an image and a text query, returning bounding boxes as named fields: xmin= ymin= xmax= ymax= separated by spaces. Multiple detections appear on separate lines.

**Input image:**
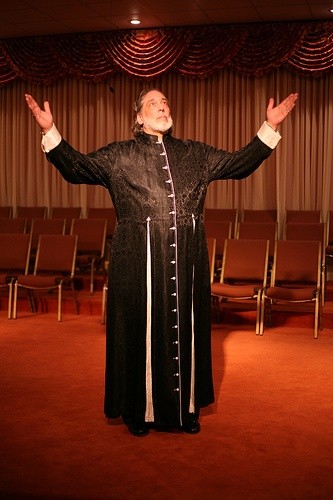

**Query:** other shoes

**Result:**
xmin=178 ymin=421 xmax=200 ymax=433
xmin=126 ymin=417 xmax=149 ymax=435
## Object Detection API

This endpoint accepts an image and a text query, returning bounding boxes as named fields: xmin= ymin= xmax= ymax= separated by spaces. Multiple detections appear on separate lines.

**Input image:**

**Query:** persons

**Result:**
xmin=24 ymin=90 xmax=298 ymax=437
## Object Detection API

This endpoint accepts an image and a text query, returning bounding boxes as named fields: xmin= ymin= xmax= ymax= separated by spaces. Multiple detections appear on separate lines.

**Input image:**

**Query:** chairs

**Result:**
xmin=0 ymin=205 xmax=333 ymax=338
